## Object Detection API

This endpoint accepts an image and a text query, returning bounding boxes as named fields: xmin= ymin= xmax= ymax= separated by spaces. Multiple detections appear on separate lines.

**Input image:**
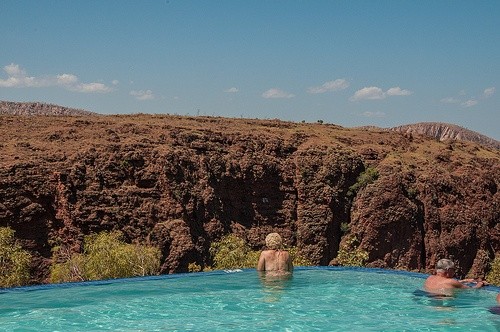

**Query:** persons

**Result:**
xmin=257 ymin=233 xmax=294 ymax=278
xmin=421 ymin=259 xmax=492 ymax=296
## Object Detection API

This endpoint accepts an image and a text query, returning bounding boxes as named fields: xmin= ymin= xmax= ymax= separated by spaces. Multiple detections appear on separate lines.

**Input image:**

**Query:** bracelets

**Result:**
xmin=480 ymin=282 xmax=484 ymax=286
